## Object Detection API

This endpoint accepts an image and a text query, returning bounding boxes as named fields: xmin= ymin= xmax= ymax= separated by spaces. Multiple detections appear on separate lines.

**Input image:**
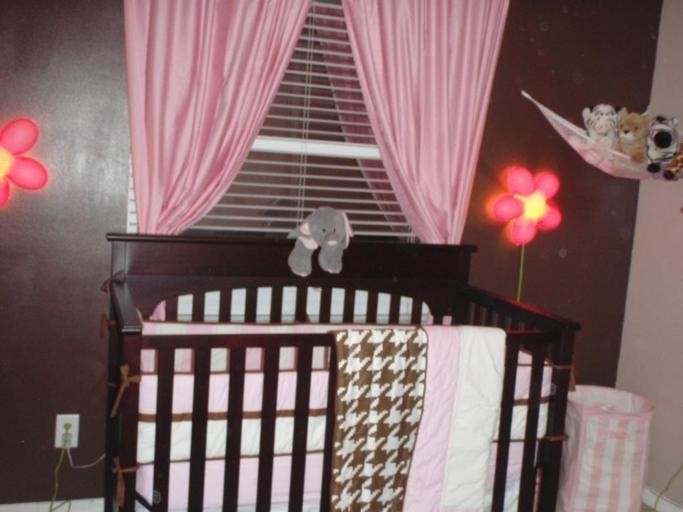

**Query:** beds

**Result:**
xmin=106 ymin=233 xmax=581 ymax=512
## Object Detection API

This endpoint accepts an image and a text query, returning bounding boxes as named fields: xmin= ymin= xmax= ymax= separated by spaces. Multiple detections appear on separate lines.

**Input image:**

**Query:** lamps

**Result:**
xmin=493 ymin=167 xmax=560 ymax=306
xmin=0 ymin=118 xmax=49 ymax=207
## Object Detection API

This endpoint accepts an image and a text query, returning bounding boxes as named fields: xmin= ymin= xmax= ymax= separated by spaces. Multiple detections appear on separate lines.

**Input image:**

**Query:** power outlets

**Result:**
xmin=54 ymin=412 xmax=79 ymax=451
